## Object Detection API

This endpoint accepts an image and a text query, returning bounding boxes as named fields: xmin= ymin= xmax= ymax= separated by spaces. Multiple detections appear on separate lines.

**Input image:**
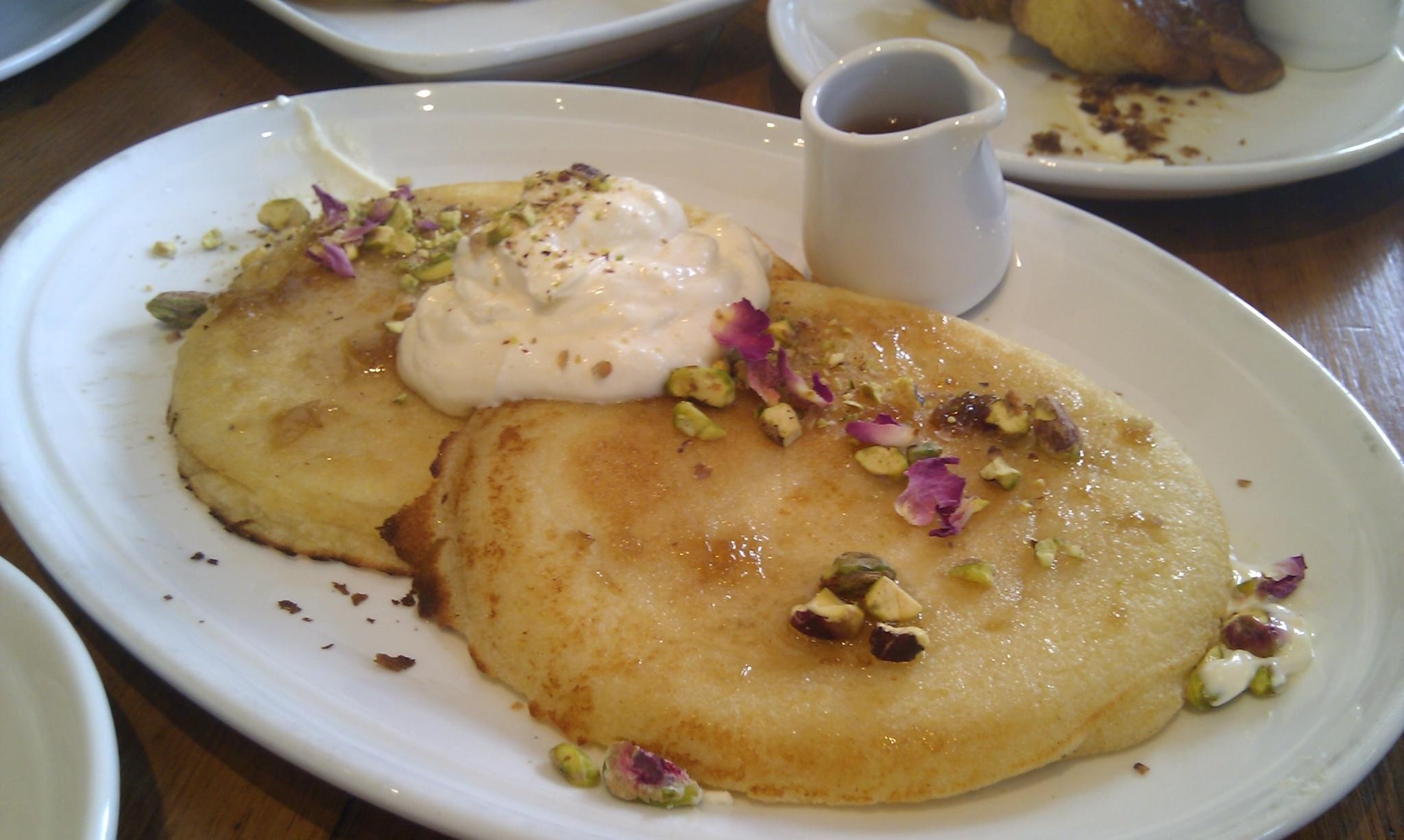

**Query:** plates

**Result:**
xmin=0 ymin=557 xmax=123 ymax=840
xmin=248 ymin=1 xmax=756 ymax=88
xmin=765 ymin=0 xmax=1404 ymax=207
xmin=0 ymin=82 xmax=1403 ymax=840
xmin=1 ymin=0 xmax=130 ymax=87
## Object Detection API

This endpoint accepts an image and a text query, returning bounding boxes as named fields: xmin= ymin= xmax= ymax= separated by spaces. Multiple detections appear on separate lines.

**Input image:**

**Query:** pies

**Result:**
xmin=161 ymin=177 xmax=1238 ymax=805
xmin=1003 ymin=0 xmax=1284 ymax=94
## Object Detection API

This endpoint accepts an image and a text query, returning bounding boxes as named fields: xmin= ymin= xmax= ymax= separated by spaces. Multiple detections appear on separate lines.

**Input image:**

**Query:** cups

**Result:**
xmin=798 ymin=35 xmax=1021 ymax=324
xmin=1243 ymin=0 xmax=1404 ymax=74
